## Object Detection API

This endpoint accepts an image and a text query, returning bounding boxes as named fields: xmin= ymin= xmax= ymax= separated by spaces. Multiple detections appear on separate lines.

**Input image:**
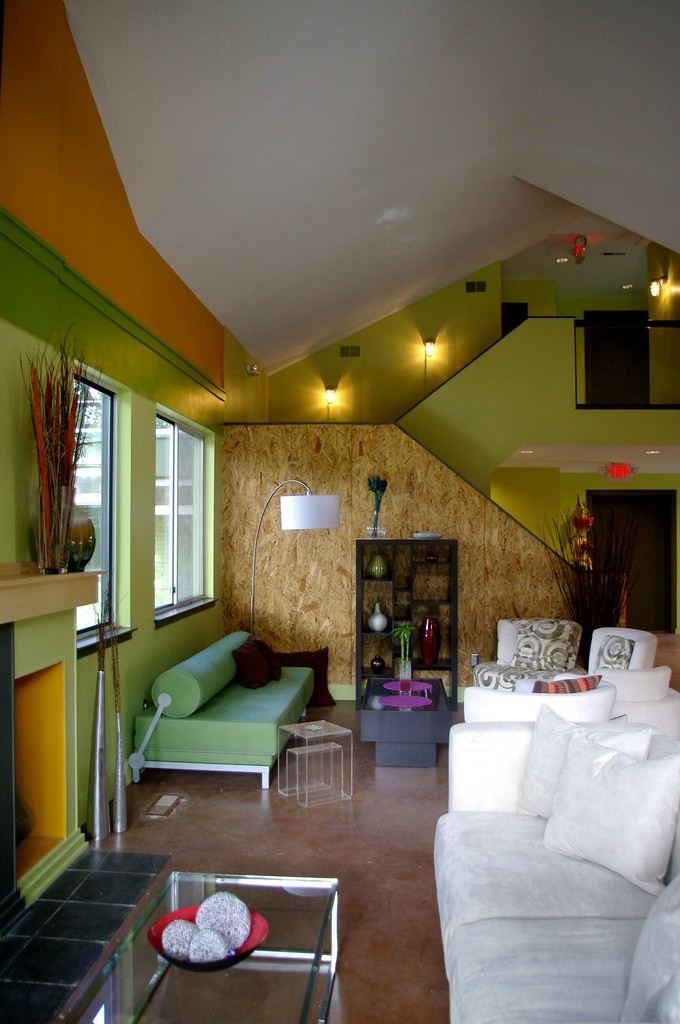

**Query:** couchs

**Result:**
xmin=464 ymin=627 xmax=680 ymax=739
xmin=433 ymin=722 xmax=680 ymax=1024
xmin=474 ymin=619 xmax=588 ymax=690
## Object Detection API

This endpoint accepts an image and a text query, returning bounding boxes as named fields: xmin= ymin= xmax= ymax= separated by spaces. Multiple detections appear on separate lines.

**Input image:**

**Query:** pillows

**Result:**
xmin=532 ymin=674 xmax=603 ymax=693
xmin=509 ymin=619 xmax=582 ymax=672
xmin=542 ymin=726 xmax=680 ymax=897
xmin=519 ymin=701 xmax=652 ymax=819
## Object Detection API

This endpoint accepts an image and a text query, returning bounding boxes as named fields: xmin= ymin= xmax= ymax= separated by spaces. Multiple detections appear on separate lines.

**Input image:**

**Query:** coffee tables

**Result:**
xmin=60 ymin=872 xmax=338 ymax=1024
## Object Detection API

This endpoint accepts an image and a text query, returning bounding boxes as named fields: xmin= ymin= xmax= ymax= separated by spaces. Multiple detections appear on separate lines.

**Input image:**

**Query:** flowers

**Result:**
xmin=361 ymin=540 xmax=381 ymax=555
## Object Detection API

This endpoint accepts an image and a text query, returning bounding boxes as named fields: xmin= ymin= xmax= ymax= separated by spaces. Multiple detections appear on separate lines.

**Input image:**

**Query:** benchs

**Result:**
xmin=128 ymin=631 xmax=314 ymax=790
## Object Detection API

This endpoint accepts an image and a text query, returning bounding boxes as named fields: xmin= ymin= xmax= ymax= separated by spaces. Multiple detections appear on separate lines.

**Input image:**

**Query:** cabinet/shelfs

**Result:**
xmin=355 ymin=540 xmax=459 ymax=710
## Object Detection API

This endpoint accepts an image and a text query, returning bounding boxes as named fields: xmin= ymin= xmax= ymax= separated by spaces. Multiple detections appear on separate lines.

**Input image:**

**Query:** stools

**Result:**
xmin=276 ymin=719 xmax=353 ymax=807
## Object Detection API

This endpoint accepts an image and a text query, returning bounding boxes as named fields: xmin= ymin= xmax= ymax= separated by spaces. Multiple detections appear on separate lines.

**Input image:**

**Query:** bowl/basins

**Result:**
xmin=147 ymin=905 xmax=270 ymax=974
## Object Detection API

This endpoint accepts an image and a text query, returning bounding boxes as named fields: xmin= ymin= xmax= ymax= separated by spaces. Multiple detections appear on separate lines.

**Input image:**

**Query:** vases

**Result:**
xmin=85 ymin=671 xmax=127 ymax=840
xmin=367 ymin=602 xmax=387 ymax=632
xmin=421 ymin=617 xmax=440 ymax=665
xmin=366 ymin=554 xmax=388 ymax=578
xmin=399 ymin=662 xmax=412 ymax=692
xmin=370 ymin=655 xmax=384 ymax=674
xmin=372 ymin=511 xmax=378 ymax=537
xmin=28 ymin=505 xmax=96 ymax=573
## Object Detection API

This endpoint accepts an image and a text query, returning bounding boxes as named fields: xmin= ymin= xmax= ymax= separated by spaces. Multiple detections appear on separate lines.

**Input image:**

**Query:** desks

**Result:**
xmin=360 ymin=677 xmax=453 ymax=768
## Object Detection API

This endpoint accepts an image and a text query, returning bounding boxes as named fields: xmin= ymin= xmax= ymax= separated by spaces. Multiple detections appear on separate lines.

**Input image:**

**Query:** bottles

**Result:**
xmin=420 ymin=617 xmax=441 ymax=665
xmin=368 ymin=603 xmax=387 ymax=632
xmin=370 ymin=655 xmax=385 ymax=674
xmin=28 ymin=486 xmax=96 ymax=575
xmin=366 ymin=546 xmax=387 ymax=579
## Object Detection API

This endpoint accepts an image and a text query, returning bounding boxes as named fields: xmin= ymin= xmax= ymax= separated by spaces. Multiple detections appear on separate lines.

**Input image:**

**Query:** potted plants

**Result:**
xmin=18 ymin=315 xmax=103 ymax=576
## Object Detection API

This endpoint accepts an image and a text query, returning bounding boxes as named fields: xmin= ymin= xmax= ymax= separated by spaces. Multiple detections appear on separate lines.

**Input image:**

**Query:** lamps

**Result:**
xmin=324 ymin=385 xmax=337 ymax=404
xmin=649 ymin=275 xmax=668 ymax=296
xmin=422 ymin=336 xmax=435 ymax=357
xmin=248 ymin=480 xmax=341 ymax=639
xmin=598 ymin=464 xmax=641 ymax=482
